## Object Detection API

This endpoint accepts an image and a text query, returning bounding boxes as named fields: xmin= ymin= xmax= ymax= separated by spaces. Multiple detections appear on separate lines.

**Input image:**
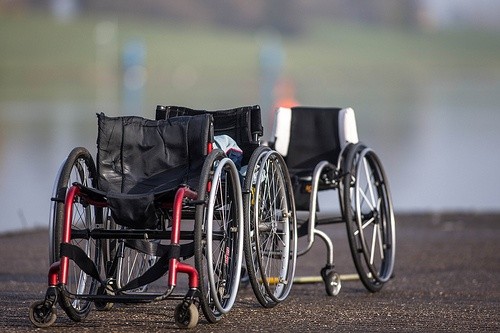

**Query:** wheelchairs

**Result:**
xmin=222 ymin=105 xmax=397 ymax=295
xmin=100 ymin=103 xmax=297 ymax=310
xmin=29 ymin=111 xmax=242 ymax=330
